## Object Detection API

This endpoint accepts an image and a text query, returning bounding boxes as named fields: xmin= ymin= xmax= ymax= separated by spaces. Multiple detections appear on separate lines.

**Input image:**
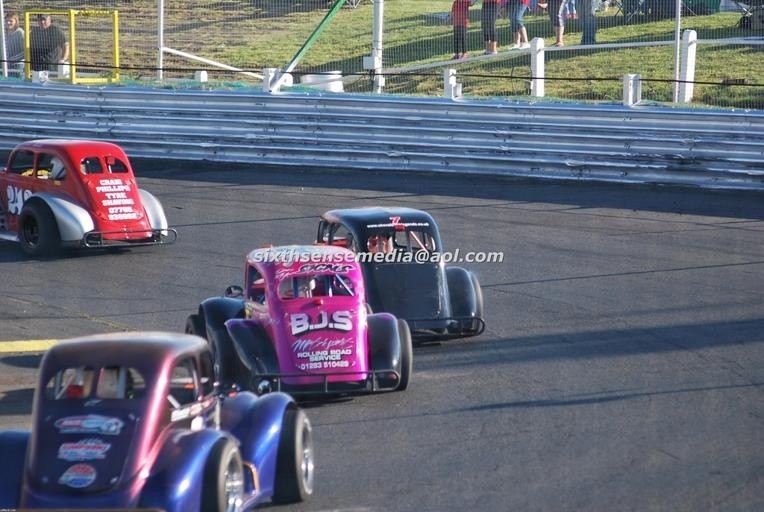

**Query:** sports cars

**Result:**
xmin=312 ymin=206 xmax=485 ymax=343
xmin=0 ymin=138 xmax=178 ymax=256
xmin=183 ymin=243 xmax=412 ymax=398
xmin=0 ymin=330 xmax=313 ymax=511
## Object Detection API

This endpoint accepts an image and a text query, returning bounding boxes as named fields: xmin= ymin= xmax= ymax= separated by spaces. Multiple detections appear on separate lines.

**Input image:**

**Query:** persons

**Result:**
xmin=574 ymin=0 xmax=600 ymax=45
xmin=443 ymin=0 xmax=473 ymax=61
xmin=505 ymin=0 xmax=531 ymax=51
xmin=28 ymin=13 xmax=68 ymax=77
xmin=497 ymin=0 xmax=618 ymax=22
xmin=0 ymin=12 xmax=25 ymax=79
xmin=544 ymin=0 xmax=569 ymax=47
xmin=46 ymin=155 xmax=66 ymax=182
xmin=478 ymin=0 xmax=499 ymax=55
